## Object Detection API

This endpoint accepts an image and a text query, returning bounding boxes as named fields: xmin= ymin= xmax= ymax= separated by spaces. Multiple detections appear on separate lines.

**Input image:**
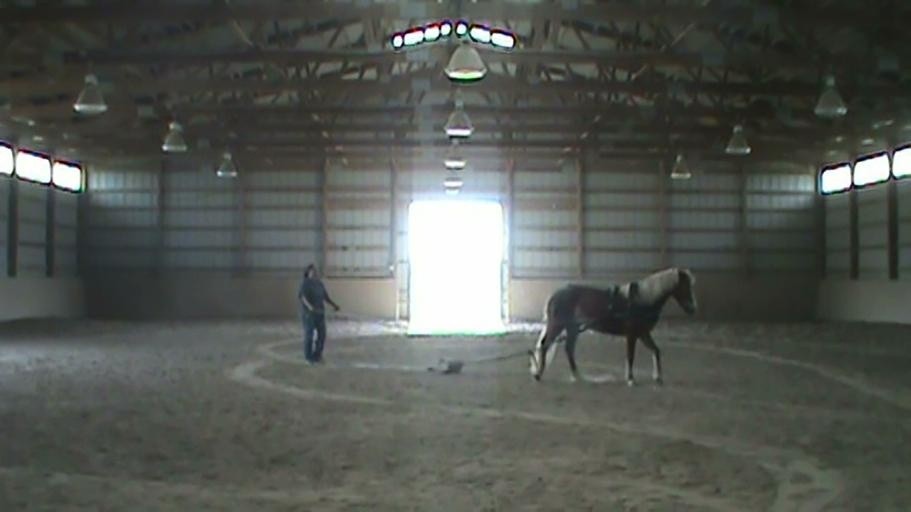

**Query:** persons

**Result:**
xmin=296 ymin=264 xmax=342 ymax=365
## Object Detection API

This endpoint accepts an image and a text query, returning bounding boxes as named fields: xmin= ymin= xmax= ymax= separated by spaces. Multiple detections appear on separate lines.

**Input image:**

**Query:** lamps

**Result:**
xmin=160 ymin=118 xmax=189 ymax=154
xmin=723 ymin=121 xmax=752 ymax=155
xmin=213 ymin=142 xmax=237 ymax=180
xmin=667 ymin=152 xmax=692 ymax=180
xmin=441 ymin=38 xmax=489 ymax=171
xmin=814 ymin=71 xmax=847 ymax=120
xmin=73 ymin=70 xmax=108 ymax=115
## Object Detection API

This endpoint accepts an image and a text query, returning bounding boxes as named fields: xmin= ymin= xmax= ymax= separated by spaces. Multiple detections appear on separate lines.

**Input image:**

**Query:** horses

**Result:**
xmin=530 ymin=264 xmax=700 ymax=387
